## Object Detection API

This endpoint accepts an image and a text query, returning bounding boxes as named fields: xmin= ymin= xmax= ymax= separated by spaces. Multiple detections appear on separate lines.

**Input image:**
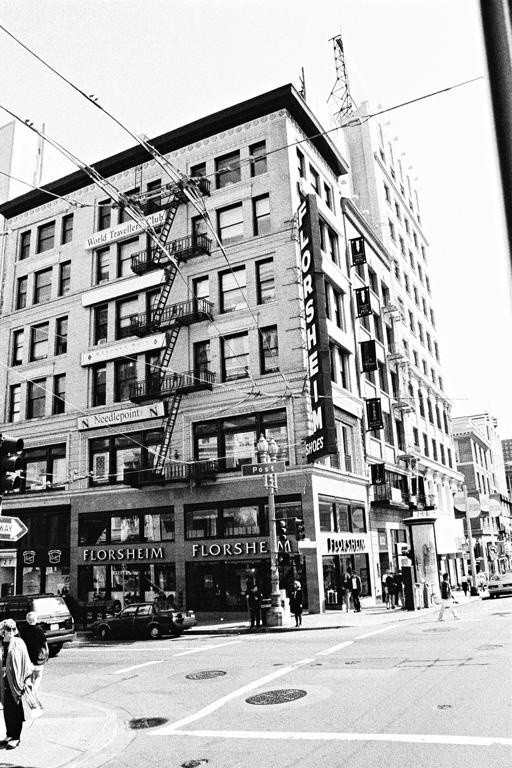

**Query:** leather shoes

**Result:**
xmin=0 ymin=737 xmax=21 ymax=750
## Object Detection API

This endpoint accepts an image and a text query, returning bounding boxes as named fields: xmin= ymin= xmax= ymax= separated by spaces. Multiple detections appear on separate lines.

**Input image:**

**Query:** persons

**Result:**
xmin=382 ymin=570 xmax=407 ymax=611
xmin=461 ymin=574 xmax=469 ymax=597
xmin=247 ymin=584 xmax=264 ymax=630
xmin=289 ymin=578 xmax=303 ymax=627
xmin=340 ymin=571 xmax=350 ymax=613
xmin=348 ymin=570 xmax=362 ymax=613
xmin=23 ymin=610 xmax=51 ymax=704
xmin=437 ymin=572 xmax=462 ymax=620
xmin=0 ymin=616 xmax=35 ymax=749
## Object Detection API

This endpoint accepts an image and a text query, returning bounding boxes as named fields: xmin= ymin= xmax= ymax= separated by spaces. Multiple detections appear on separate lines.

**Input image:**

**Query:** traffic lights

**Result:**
xmin=1 ymin=434 xmax=26 ymax=495
xmin=280 ymin=520 xmax=287 ymax=542
xmin=295 ymin=519 xmax=305 ymax=540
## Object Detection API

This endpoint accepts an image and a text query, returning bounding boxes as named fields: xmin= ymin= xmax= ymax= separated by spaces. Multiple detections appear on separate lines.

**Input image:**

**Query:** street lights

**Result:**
xmin=241 ymin=432 xmax=291 ymax=626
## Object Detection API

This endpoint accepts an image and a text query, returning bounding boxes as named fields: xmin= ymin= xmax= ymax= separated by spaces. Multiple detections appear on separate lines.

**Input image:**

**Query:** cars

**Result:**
xmin=87 ymin=602 xmax=197 ymax=641
xmin=1 ymin=593 xmax=77 ymax=658
xmin=488 ymin=572 xmax=512 ymax=599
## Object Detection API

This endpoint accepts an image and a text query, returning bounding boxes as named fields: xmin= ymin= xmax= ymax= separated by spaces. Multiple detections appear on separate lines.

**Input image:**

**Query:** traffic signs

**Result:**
xmin=0 ymin=517 xmax=26 ymax=543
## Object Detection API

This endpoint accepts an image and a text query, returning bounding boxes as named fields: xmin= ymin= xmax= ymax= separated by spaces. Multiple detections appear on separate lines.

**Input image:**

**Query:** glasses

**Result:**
xmin=6 ymin=626 xmax=17 ymax=632
